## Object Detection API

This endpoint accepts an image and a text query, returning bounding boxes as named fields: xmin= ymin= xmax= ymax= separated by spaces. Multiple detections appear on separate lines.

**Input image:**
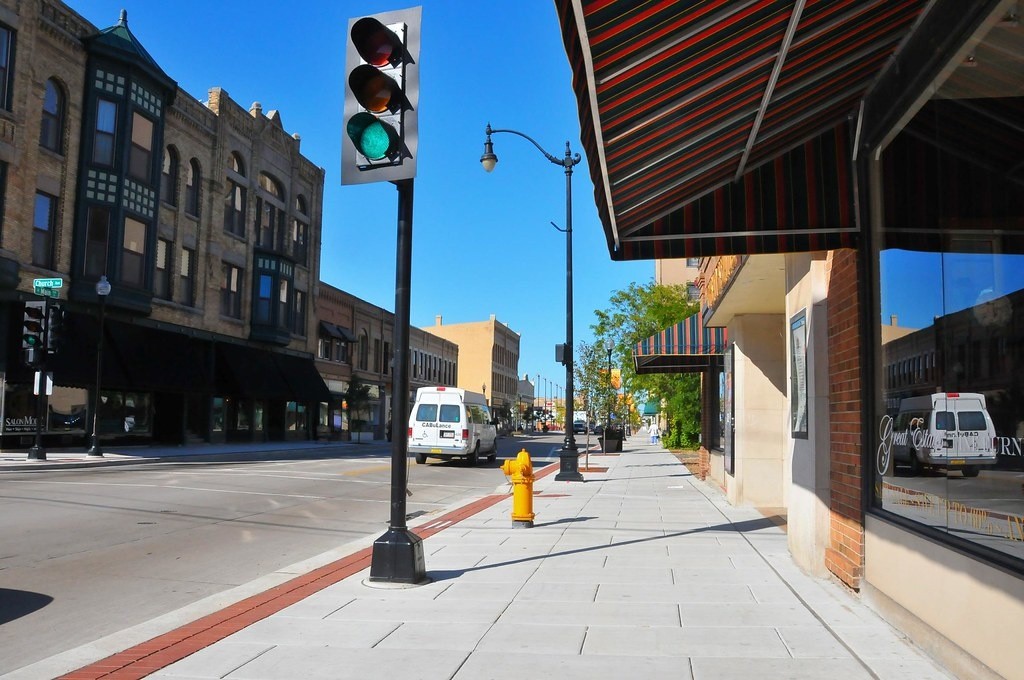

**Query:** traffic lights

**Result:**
xmin=340 ymin=5 xmax=422 ymax=186
xmin=22 ymin=300 xmax=47 ymax=349
xmin=47 ymin=304 xmax=65 ymax=354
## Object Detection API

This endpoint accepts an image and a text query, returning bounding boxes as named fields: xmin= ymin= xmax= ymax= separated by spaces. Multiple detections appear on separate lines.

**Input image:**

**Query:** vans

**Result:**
xmin=892 ymin=390 xmax=999 ymax=477
xmin=407 ymin=385 xmax=500 ymax=466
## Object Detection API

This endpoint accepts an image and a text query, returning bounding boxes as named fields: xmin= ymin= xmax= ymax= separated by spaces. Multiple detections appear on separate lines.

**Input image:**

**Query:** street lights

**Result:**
xmin=605 ymin=336 xmax=614 ymax=429
xmin=479 ymin=120 xmax=583 ymax=482
xmin=88 ymin=276 xmax=112 ymax=457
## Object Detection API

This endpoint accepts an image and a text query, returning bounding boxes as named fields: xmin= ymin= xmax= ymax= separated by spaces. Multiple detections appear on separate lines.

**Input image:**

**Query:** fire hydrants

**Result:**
xmin=500 ymin=448 xmax=535 ymax=529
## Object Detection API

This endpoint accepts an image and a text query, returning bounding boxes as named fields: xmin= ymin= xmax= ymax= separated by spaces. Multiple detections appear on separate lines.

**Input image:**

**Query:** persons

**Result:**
xmin=648 ymin=421 xmax=659 ymax=445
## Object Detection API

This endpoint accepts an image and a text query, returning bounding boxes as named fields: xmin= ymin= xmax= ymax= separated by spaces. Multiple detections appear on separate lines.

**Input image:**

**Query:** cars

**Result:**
xmin=594 ymin=425 xmax=603 ymax=435
xmin=573 ymin=419 xmax=587 ymax=434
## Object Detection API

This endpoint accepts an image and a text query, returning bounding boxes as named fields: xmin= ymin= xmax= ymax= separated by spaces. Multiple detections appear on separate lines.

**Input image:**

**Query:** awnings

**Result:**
xmin=554 ymin=0 xmax=1023 ymax=259
xmin=12 ymin=302 xmax=335 ymax=402
xmin=318 ymin=321 xmax=357 ymax=344
xmin=633 ymin=312 xmax=731 ymax=376
